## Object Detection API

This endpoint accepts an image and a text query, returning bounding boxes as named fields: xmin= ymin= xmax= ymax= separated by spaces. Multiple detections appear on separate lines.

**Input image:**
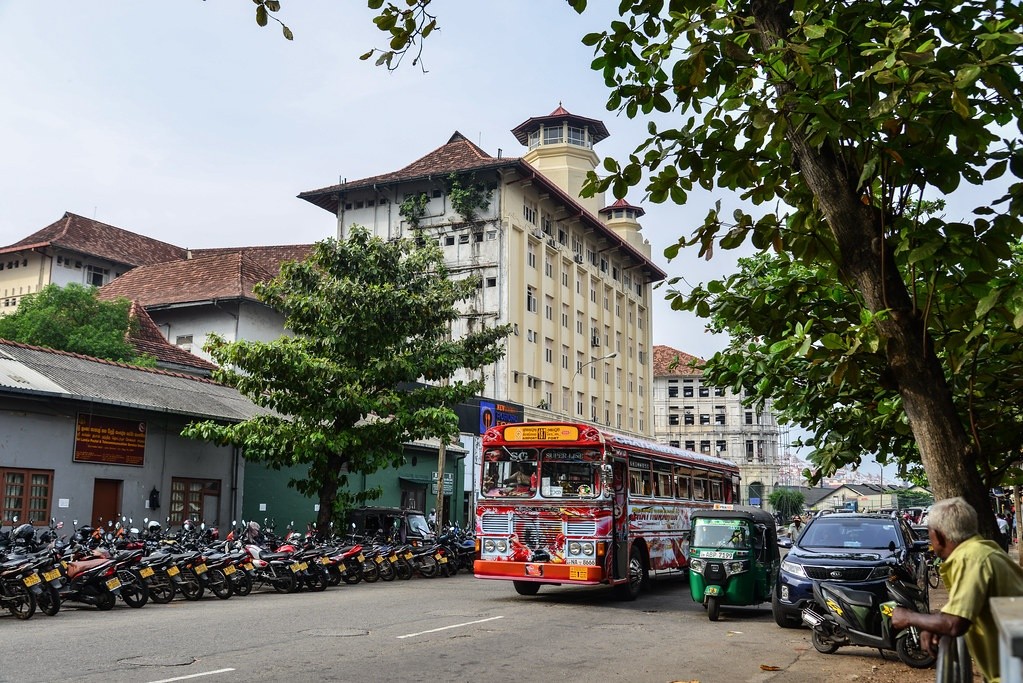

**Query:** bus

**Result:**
xmin=472 ymin=421 xmax=742 ymax=600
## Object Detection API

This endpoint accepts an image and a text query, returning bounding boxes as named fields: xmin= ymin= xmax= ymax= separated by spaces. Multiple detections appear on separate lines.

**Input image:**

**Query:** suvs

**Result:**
xmin=772 ymin=506 xmax=924 ymax=628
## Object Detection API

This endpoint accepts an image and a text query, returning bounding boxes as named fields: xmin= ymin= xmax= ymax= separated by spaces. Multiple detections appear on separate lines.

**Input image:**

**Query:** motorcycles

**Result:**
xmin=803 ymin=542 xmax=937 ymax=670
xmin=1 ymin=491 xmax=481 ymax=622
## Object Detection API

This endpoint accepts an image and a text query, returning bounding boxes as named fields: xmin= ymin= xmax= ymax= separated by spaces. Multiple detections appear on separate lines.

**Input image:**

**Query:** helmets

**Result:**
xmin=13 ymin=523 xmax=34 ymax=542
xmin=288 ymin=532 xmax=302 ymax=546
xmin=249 ymin=521 xmax=260 ymax=535
xmin=148 ymin=520 xmax=162 ymax=533
xmin=431 ymin=508 xmax=435 ymax=515
xmin=181 ymin=520 xmax=195 ymax=532
xmin=346 ymin=522 xmax=357 ymax=535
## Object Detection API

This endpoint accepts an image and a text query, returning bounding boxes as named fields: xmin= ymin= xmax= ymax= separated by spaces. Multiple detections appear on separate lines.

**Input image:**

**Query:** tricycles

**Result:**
xmin=682 ymin=506 xmax=781 ymax=621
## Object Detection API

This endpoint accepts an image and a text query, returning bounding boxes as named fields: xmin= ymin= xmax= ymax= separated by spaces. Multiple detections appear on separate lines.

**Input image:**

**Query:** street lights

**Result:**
xmin=571 ymin=353 xmax=617 ymax=420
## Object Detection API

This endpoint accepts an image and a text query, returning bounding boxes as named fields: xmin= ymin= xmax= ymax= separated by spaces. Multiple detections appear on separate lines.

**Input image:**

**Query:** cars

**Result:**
xmin=774 ymin=490 xmax=949 ymax=560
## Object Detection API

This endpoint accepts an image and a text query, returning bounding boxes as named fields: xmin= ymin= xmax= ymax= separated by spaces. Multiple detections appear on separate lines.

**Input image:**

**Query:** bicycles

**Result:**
xmin=925 ymin=548 xmax=944 ymax=589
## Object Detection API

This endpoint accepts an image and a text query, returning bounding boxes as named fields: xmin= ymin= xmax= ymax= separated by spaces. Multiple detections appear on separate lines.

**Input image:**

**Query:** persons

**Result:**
xmin=861 ymin=500 xmax=1021 ymax=552
xmin=428 ymin=507 xmax=436 ymax=526
xmin=891 ymin=497 xmax=1022 ymax=682
xmin=787 ymin=515 xmax=807 ymax=542
xmin=503 ymin=463 xmax=533 ymax=483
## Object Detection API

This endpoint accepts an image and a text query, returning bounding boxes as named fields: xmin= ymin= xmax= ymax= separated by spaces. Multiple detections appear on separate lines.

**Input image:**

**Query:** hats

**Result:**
xmin=794 ymin=516 xmax=801 ymax=522
